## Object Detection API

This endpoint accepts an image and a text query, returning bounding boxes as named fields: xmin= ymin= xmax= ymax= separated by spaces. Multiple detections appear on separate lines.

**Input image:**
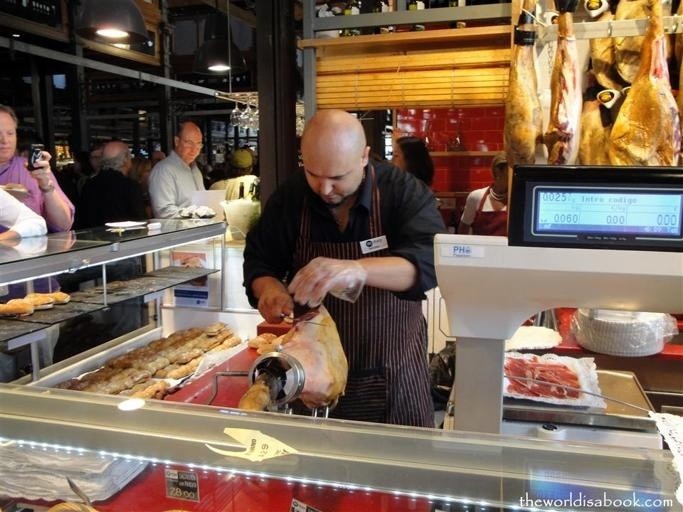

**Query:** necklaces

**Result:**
xmin=489 ymin=187 xmax=506 ymax=200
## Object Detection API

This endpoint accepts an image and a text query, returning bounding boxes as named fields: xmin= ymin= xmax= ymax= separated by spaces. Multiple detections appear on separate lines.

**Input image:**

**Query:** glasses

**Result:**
xmin=180 ymin=138 xmax=205 ymax=149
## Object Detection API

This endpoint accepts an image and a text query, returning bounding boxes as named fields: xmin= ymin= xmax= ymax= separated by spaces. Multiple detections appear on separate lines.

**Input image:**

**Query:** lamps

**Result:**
xmin=76 ymin=2 xmax=151 ymax=43
xmin=193 ymin=2 xmax=246 ymax=73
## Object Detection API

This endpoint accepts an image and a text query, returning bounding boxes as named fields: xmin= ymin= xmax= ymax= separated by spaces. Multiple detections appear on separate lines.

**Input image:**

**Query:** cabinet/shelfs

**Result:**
xmin=1 ymin=216 xmax=226 ymax=388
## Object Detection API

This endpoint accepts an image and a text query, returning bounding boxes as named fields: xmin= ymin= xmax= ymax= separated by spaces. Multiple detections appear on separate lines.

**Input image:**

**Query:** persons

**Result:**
xmin=393 ymin=136 xmax=433 ymax=188
xmin=457 ymin=155 xmax=508 ymax=237
xmin=0 ymin=98 xmax=76 ymax=382
xmin=62 ymin=122 xmax=259 ymax=319
xmin=244 ymin=110 xmax=447 ymax=427
xmin=0 ymin=185 xmax=48 ymax=241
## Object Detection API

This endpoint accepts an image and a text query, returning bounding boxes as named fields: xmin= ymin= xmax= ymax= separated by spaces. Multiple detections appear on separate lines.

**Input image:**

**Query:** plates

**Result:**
xmin=105 ymin=220 xmax=147 ymax=228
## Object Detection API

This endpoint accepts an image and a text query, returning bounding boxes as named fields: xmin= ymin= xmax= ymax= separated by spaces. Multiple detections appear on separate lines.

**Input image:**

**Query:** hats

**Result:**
xmin=231 ymin=150 xmax=253 ymax=169
xmin=495 ymin=156 xmax=506 ymax=168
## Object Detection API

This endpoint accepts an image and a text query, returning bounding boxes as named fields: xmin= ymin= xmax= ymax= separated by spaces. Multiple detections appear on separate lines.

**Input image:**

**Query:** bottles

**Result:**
xmin=239 ymin=182 xmax=244 ymax=198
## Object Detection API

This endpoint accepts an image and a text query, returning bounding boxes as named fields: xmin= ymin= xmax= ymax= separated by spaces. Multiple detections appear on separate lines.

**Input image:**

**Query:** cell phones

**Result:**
xmin=27 ymin=142 xmax=44 ymax=170
